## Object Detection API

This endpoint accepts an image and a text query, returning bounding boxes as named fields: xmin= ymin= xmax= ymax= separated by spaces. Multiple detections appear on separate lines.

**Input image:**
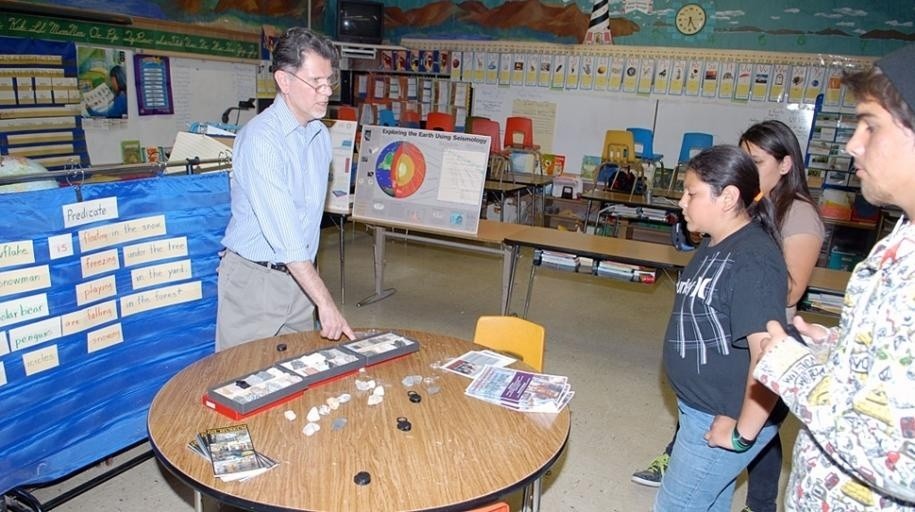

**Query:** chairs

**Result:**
xmin=336 ymin=109 xmax=713 ymax=201
xmin=473 ymin=502 xmax=508 ymax=512
xmin=474 ymin=315 xmax=546 ymax=373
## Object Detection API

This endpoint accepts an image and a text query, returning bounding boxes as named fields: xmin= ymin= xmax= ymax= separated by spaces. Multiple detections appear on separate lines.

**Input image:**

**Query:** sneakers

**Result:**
xmin=632 ymin=453 xmax=671 ymax=487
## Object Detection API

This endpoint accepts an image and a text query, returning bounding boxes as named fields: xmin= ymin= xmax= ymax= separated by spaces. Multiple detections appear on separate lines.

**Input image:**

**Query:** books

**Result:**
xmin=187 ymin=423 xmax=279 ymax=482
xmin=807 ymin=176 xmax=880 ymax=230
xmin=807 ymin=292 xmax=844 ymax=314
xmin=541 ymin=249 xmax=657 ymax=284
xmin=611 ymin=205 xmax=668 ymax=223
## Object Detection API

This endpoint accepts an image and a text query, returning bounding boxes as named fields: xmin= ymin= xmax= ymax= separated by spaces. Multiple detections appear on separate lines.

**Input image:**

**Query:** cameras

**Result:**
xmin=782 ymin=324 xmax=808 ymax=347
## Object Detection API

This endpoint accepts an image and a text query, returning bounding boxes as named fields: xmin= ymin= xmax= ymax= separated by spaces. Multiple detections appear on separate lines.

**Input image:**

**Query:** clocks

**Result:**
xmin=675 ymin=4 xmax=706 ymax=36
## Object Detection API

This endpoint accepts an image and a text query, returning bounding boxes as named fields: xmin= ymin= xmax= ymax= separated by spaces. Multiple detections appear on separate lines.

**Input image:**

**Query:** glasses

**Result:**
xmin=273 ymin=68 xmax=340 ymax=93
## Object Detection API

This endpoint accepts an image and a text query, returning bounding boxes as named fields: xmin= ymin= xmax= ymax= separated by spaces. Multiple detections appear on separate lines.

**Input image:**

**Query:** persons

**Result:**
xmin=631 ymin=118 xmax=826 ymax=512
xmin=653 ymin=145 xmax=796 ymax=512
xmin=215 ymin=26 xmax=356 ymax=341
xmin=751 ymin=44 xmax=915 ymax=512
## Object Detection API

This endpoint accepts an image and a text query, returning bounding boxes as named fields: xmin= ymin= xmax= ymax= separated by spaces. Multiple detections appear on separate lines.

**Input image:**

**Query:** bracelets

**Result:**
xmin=732 ymin=423 xmax=755 ymax=452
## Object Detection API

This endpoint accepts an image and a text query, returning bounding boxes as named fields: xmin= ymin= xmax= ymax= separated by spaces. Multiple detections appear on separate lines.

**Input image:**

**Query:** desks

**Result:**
xmin=147 ymin=330 xmax=571 ymax=511
xmin=796 ymin=266 xmax=853 ymax=318
xmin=484 ymin=181 xmax=526 ymax=223
xmin=493 ymin=173 xmax=554 ymax=227
xmin=499 ymin=226 xmax=692 ymax=318
xmin=313 ymin=207 xmax=352 ymax=305
xmin=583 ymin=190 xmax=685 ymax=238
xmin=346 ymin=214 xmax=528 ymax=319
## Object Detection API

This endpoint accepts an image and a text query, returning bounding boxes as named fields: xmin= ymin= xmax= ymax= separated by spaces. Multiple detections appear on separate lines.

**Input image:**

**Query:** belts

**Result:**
xmin=235 ymin=252 xmax=288 ymax=272
